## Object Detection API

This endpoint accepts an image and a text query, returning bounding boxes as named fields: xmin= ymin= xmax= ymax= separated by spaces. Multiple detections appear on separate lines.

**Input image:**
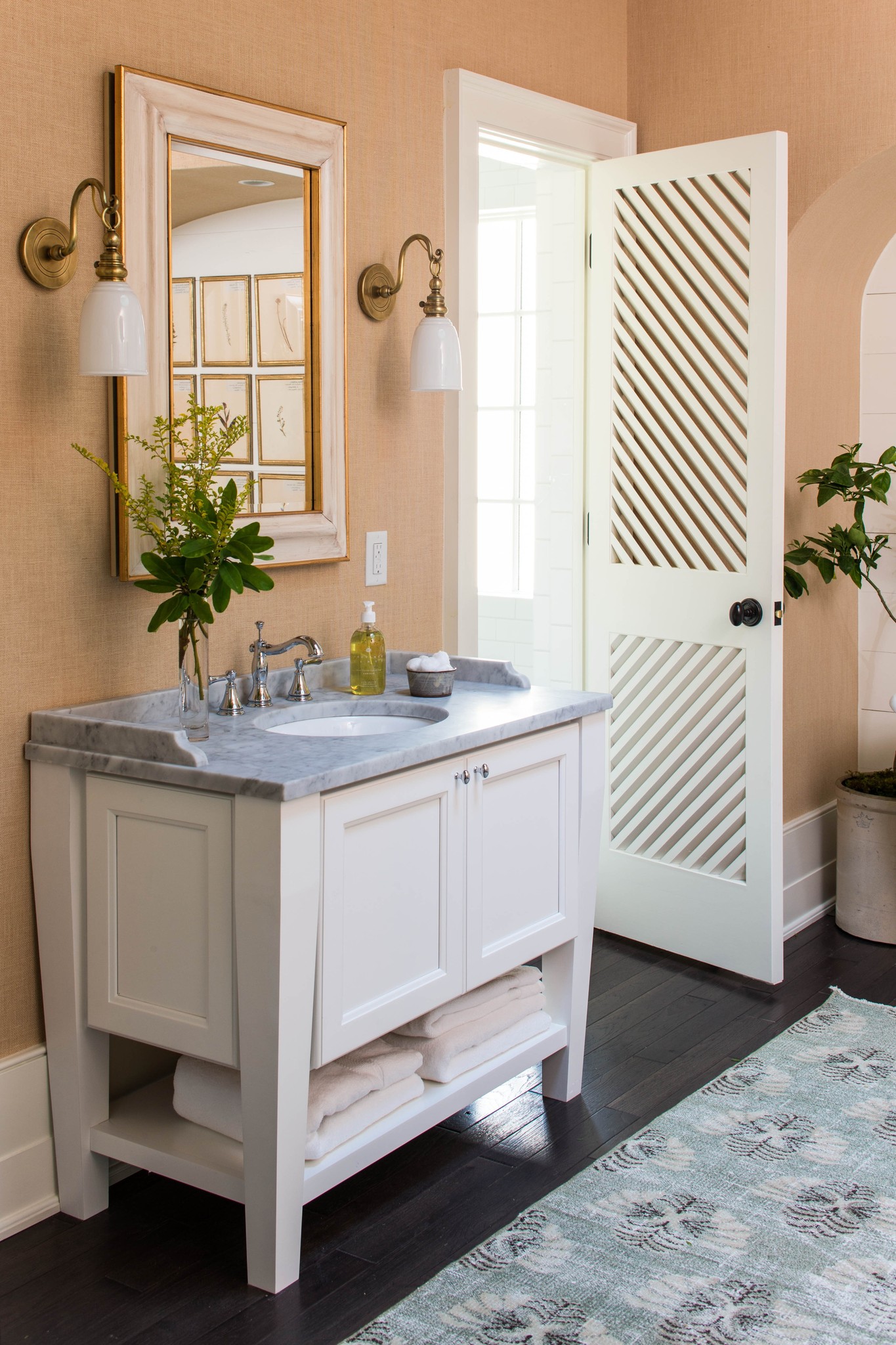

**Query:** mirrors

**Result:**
xmin=111 ymin=59 xmax=350 ymax=582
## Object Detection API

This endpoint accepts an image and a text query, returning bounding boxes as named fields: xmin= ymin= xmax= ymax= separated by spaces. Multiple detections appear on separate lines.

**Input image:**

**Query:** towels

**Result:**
xmin=391 ymin=963 xmax=548 ymax=1039
xmin=390 ymin=992 xmax=559 ymax=1082
xmin=172 ymin=1038 xmax=424 ymax=1162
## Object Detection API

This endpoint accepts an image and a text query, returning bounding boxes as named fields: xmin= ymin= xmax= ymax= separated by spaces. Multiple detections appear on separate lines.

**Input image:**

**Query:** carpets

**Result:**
xmin=334 ymin=980 xmax=896 ymax=1344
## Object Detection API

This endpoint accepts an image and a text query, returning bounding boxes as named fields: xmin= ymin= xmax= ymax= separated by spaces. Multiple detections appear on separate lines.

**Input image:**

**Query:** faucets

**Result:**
xmin=249 ymin=621 xmax=324 ymax=708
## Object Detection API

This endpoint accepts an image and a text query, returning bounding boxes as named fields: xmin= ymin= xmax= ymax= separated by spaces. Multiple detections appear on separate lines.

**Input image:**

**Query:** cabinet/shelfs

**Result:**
xmin=317 ymin=719 xmax=580 ymax=1062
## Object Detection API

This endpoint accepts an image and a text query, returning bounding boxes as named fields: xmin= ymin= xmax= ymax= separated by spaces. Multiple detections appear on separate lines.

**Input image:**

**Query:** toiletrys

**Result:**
xmin=350 ymin=601 xmax=387 ymax=695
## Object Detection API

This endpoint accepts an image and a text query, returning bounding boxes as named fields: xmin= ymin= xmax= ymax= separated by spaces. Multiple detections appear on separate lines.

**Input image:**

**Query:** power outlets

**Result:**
xmin=366 ymin=531 xmax=387 ymax=587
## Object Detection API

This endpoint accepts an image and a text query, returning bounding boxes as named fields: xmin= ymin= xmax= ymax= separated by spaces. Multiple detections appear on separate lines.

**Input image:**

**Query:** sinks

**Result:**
xmin=252 ymin=700 xmax=449 ymax=739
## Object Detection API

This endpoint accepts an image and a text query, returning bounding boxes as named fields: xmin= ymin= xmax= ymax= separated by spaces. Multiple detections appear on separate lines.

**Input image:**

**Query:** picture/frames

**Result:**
xmin=254 ymin=274 xmax=305 ymax=366
xmin=198 ymin=373 xmax=254 ymax=465
xmin=198 ymin=277 xmax=253 ymax=366
xmin=173 ymin=374 xmax=200 ymax=464
xmin=199 ymin=470 xmax=254 ymax=513
xmin=258 ymin=472 xmax=304 ymax=513
xmin=173 ymin=277 xmax=198 ymax=368
xmin=256 ymin=374 xmax=305 ymax=466
xmin=256 ymin=373 xmax=308 ymax=466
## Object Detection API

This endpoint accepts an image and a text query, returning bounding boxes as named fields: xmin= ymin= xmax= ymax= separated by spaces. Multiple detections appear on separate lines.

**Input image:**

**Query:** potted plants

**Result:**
xmin=69 ymin=383 xmax=282 ymax=740
xmin=782 ymin=439 xmax=896 ymax=950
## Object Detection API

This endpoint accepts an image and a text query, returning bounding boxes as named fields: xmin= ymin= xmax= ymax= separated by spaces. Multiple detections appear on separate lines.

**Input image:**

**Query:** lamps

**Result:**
xmin=357 ymin=232 xmax=465 ymax=395
xmin=16 ymin=177 xmax=153 ymax=378
